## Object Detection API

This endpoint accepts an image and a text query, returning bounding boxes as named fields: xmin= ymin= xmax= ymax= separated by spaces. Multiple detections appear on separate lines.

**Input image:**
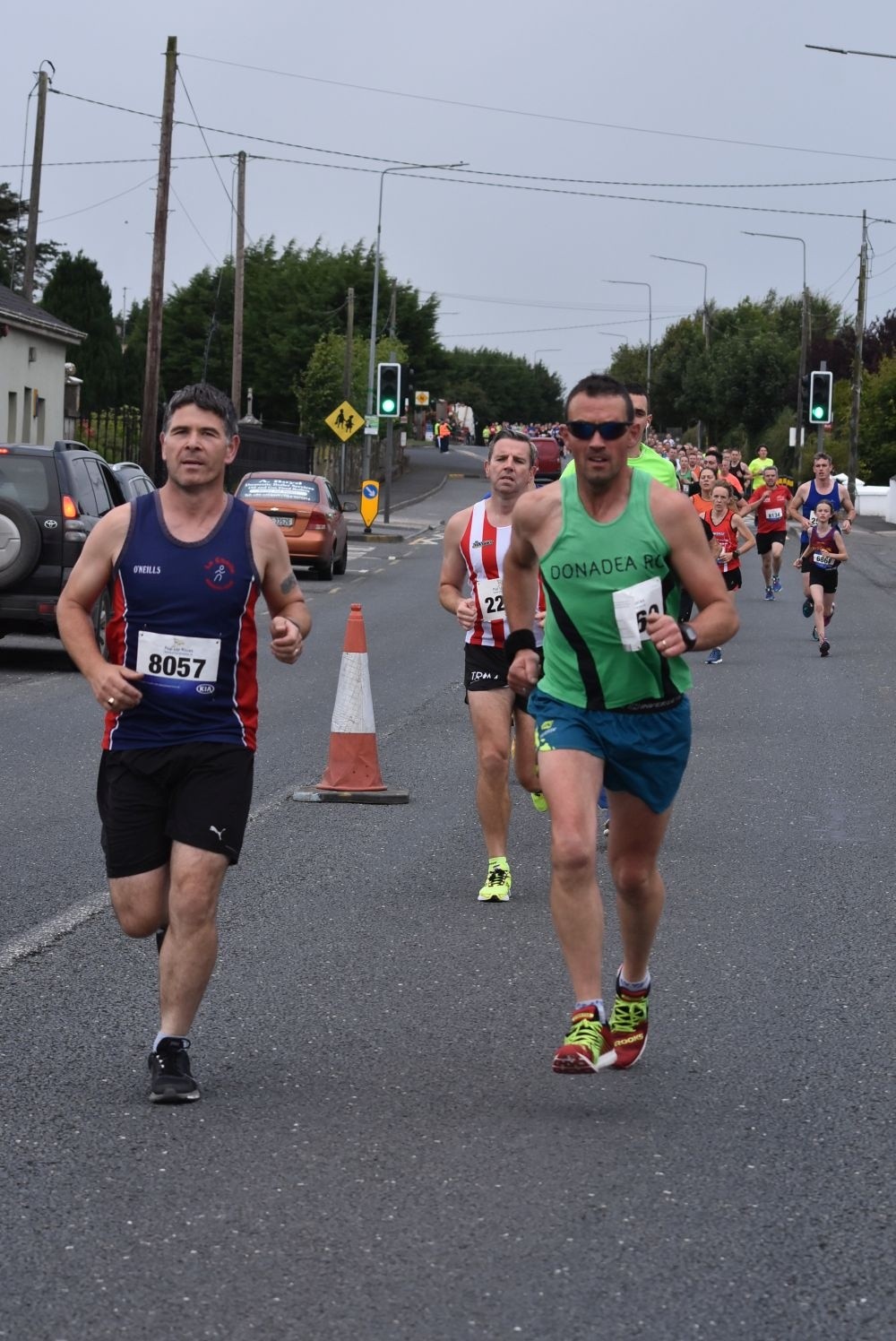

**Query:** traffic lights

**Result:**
xmin=400 ymin=364 xmax=415 ymax=417
xmin=802 ymin=373 xmax=818 ymax=430
xmin=809 ymin=371 xmax=833 ymax=424
xmin=376 ymin=362 xmax=401 ymax=417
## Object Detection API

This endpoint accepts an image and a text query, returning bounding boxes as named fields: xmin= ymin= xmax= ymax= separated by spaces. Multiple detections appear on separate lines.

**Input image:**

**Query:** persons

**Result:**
xmin=435 ymin=374 xmax=857 ymax=1080
xmin=55 ymin=387 xmax=312 ymax=1104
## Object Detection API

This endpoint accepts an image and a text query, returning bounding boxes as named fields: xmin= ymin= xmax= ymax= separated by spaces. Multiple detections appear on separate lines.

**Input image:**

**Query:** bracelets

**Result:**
xmin=504 ymin=630 xmax=537 ymax=660
xmin=801 ymin=555 xmax=805 ymax=561
xmin=846 ymin=519 xmax=852 ymax=525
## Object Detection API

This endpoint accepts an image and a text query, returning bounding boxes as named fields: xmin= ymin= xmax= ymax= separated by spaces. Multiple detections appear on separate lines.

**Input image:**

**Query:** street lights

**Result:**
xmin=363 ymin=160 xmax=471 ymax=482
xmin=599 ymin=331 xmax=629 ymax=347
xmin=533 ymin=348 xmax=563 ymax=365
xmin=651 ymin=254 xmax=708 ymax=449
xmin=741 ymin=230 xmax=808 ymax=496
xmin=848 ymin=219 xmax=892 ymax=507
xmin=606 ymin=279 xmax=652 ymax=439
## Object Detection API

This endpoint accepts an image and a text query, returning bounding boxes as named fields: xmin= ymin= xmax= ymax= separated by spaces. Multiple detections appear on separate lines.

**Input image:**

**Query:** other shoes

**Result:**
xmin=604 ymin=821 xmax=610 ymax=836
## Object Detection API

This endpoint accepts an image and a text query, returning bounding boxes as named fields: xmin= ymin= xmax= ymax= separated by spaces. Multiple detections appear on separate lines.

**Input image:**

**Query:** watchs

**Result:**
xmin=677 ymin=623 xmax=697 ymax=653
xmin=732 ymin=551 xmax=739 ymax=559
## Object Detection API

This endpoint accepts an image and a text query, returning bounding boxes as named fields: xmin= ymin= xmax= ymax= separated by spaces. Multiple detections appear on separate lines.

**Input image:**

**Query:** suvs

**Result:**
xmin=108 ymin=461 xmax=157 ymax=502
xmin=0 ymin=439 xmax=128 ymax=664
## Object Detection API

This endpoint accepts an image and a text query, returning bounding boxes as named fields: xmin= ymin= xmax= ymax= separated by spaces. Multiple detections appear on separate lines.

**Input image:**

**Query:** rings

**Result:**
xmin=295 ymin=648 xmax=300 ymax=655
xmin=107 ymin=696 xmax=115 ymax=706
xmin=662 ymin=640 xmax=668 ymax=648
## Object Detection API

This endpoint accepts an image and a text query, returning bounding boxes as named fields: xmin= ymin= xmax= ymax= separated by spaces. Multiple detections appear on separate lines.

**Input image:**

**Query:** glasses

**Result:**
xmin=567 ymin=420 xmax=632 ymax=440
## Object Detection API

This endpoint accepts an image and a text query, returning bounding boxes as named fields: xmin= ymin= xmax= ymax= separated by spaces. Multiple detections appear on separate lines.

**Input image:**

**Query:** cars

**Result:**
xmin=530 ymin=436 xmax=564 ymax=480
xmin=233 ymin=470 xmax=358 ymax=581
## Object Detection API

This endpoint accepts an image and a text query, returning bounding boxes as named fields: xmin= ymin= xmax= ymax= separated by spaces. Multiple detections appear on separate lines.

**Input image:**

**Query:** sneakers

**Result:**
xmin=764 ymin=586 xmax=775 ymax=600
xmin=705 ymin=648 xmax=722 ymax=664
xmin=823 ymin=602 xmax=835 ymax=627
xmin=147 ymin=1037 xmax=201 ymax=1101
xmin=812 ymin=626 xmax=819 ymax=639
xmin=803 ymin=599 xmax=814 ymax=617
xmin=156 ymin=923 xmax=168 ymax=954
xmin=608 ymin=963 xmax=651 ymax=1069
xmin=819 ymin=632 xmax=830 ymax=657
xmin=477 ymin=863 xmax=511 ymax=901
xmin=552 ymin=1006 xmax=618 ymax=1074
xmin=531 ymin=791 xmax=548 ymax=812
xmin=772 ymin=575 xmax=782 ymax=592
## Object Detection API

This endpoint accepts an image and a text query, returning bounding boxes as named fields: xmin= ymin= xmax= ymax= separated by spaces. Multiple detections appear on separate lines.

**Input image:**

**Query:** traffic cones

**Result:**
xmin=292 ymin=604 xmax=409 ymax=805
